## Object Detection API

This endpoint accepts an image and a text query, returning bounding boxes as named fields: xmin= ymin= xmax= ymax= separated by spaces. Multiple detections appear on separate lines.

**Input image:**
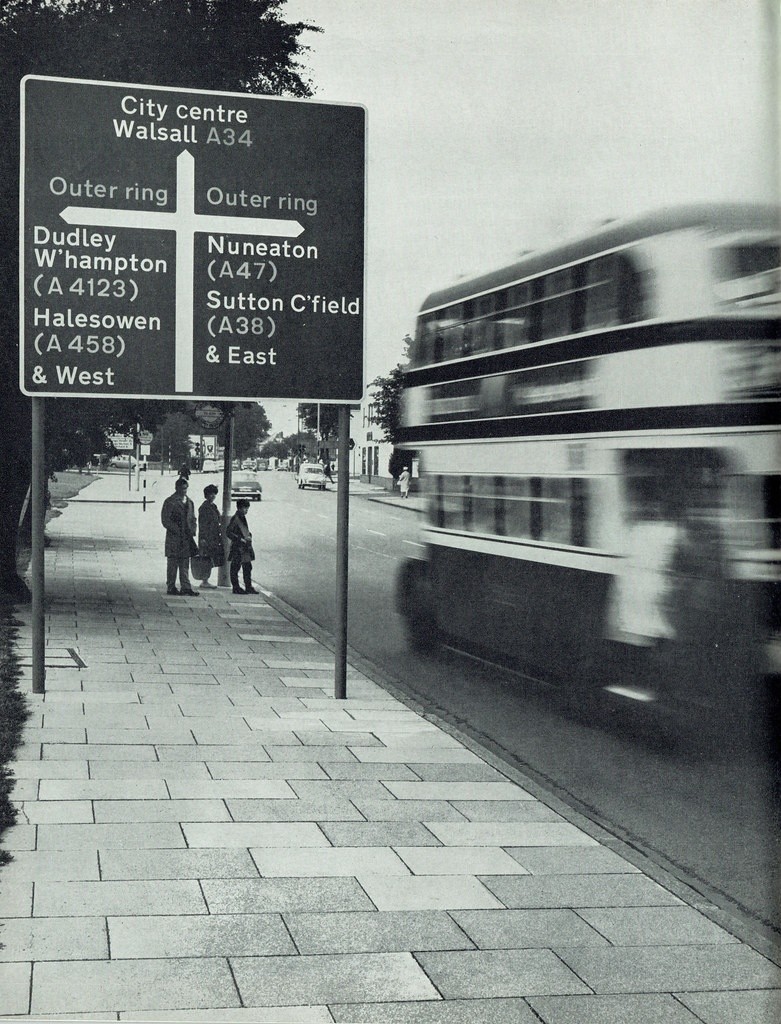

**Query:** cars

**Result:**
xmin=231 ymin=470 xmax=263 ymax=500
xmin=109 ymin=455 xmax=136 ymax=470
xmin=200 ymin=458 xmax=270 ymax=474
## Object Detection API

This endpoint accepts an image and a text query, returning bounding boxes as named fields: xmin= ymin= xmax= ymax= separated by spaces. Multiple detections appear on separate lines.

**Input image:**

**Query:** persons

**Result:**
xmin=324 ymin=460 xmax=335 ymax=484
xmin=161 ymin=479 xmax=200 ymax=596
xmin=198 ymin=485 xmax=224 ymax=589
xmin=227 ymin=499 xmax=259 ymax=594
xmin=399 ymin=467 xmax=411 ymax=498
xmin=178 ymin=463 xmax=190 ymax=480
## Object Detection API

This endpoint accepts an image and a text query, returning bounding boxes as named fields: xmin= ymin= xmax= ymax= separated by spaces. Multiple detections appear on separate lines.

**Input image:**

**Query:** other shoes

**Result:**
xmin=246 ymin=586 xmax=259 ymax=594
xmin=180 ymin=589 xmax=199 ymax=597
xmin=200 ymin=582 xmax=218 ymax=589
xmin=167 ymin=587 xmax=180 ymax=596
xmin=233 ymin=585 xmax=248 ymax=594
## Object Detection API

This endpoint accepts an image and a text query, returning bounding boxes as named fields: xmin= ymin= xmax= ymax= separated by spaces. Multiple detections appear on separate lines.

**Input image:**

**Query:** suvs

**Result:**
xmin=297 ymin=463 xmax=327 ymax=491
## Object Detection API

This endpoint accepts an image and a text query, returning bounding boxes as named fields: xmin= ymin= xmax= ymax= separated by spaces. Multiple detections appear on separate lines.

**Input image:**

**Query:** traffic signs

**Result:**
xmin=14 ymin=73 xmax=368 ymax=409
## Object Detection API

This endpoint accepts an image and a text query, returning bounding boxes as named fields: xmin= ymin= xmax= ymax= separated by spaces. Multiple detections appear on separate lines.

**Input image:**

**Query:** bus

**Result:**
xmin=389 ymin=199 xmax=781 ymax=746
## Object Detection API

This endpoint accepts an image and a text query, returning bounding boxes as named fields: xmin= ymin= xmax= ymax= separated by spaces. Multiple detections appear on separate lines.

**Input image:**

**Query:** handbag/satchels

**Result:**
xmin=191 ymin=543 xmax=213 ymax=579
